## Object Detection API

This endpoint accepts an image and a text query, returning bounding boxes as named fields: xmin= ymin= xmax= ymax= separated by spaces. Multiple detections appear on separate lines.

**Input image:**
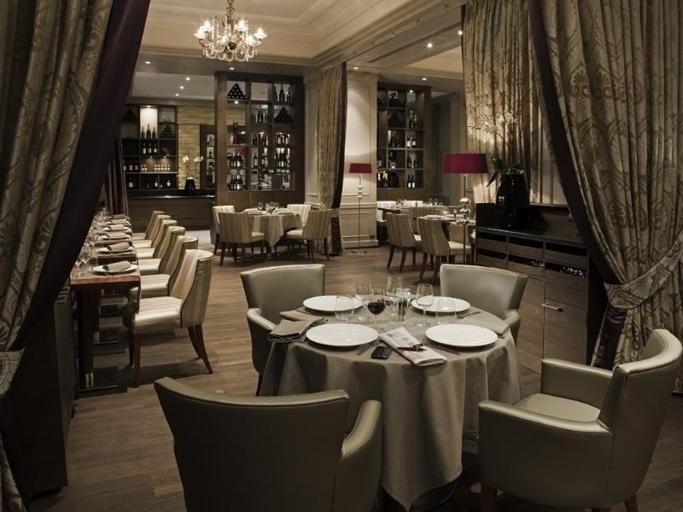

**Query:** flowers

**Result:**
xmin=466 ymin=94 xmax=531 ymax=175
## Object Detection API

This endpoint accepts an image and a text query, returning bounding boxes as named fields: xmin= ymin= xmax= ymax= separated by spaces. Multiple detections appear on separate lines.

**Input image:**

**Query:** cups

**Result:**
xmin=335 ymin=294 xmax=355 ymax=322
xmin=366 ymin=288 xmax=386 ymax=329
xmin=436 ymin=299 xmax=456 ymax=324
xmin=355 ymin=280 xmax=372 ymax=320
xmin=386 ymin=277 xmax=402 ymax=316
xmin=416 ymin=283 xmax=433 ymax=326
xmin=74 ymin=231 xmax=99 ymax=274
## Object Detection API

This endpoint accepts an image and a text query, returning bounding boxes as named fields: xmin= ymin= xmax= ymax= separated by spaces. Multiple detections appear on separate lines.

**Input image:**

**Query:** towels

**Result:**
xmin=110 ymin=219 xmax=127 ymax=224
xmin=112 ymin=214 xmax=125 ymax=219
xmin=107 ymin=241 xmax=129 ymax=251
xmin=279 ymin=310 xmax=326 ymax=326
xmin=266 ymin=318 xmax=314 ymax=343
xmin=378 ymin=326 xmax=447 ymax=367
xmin=106 ymin=232 xmax=126 ymax=238
xmin=108 ymin=224 xmax=124 ymax=230
xmin=103 ymin=260 xmax=131 ymax=272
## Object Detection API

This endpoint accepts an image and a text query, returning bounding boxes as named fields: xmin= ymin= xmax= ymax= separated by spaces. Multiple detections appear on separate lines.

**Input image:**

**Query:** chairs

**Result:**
xmin=243 ymin=263 xmax=326 ymax=374
xmin=440 ymin=264 xmax=529 ymax=347
xmin=477 ymin=328 xmax=683 ymax=511
xmin=132 ymin=215 xmax=171 ymax=248
xmin=129 ymin=249 xmax=214 ymax=388
xmin=154 ymin=376 xmax=383 ymax=511
xmin=286 ymin=209 xmax=333 ymax=263
xmin=218 ymin=212 xmax=265 ymax=267
xmin=416 ymin=216 xmax=472 ymax=286
xmin=130 ymin=235 xmax=199 ymax=303
xmin=136 ymin=219 xmax=178 ymax=259
xmin=212 ymin=205 xmax=254 ymax=257
xmin=132 ymin=225 xmax=186 ymax=276
xmin=132 ymin=210 xmax=165 ymax=240
xmin=385 ymin=212 xmax=422 ymax=273
xmin=286 ymin=204 xmax=312 ymax=228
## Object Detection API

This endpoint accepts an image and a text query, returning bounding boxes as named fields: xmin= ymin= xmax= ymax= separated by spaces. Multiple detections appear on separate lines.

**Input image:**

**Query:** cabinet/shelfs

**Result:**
xmin=473 ymin=202 xmax=608 ymax=375
xmin=123 ymin=103 xmax=179 ymax=195
xmin=128 ymin=196 xmax=215 ymax=232
xmin=214 ymin=70 xmax=305 ymax=212
xmin=206 ymin=133 xmax=215 ymax=189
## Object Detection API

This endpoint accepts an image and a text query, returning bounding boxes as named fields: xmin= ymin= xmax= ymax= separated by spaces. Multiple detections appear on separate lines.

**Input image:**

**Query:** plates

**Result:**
xmin=412 ymin=297 xmax=470 ymax=312
xmin=303 ymin=296 xmax=363 ymax=311
xmin=98 ymin=247 xmax=132 ymax=251
xmin=425 ymin=324 xmax=498 ymax=347
xmin=92 ymin=265 xmax=137 ymax=272
xmin=305 ymin=324 xmax=378 ymax=346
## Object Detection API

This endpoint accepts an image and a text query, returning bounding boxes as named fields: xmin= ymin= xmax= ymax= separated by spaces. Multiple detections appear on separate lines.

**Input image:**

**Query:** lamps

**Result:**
xmin=193 ymin=0 xmax=268 ymax=62
xmin=442 ymin=152 xmax=489 ymax=174
xmin=349 ymin=163 xmax=373 ymax=174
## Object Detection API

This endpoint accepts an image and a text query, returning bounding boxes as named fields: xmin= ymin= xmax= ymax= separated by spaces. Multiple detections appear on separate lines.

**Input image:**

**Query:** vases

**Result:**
xmin=495 ymin=175 xmax=532 ymax=231
xmin=182 ymin=155 xmax=204 ymax=196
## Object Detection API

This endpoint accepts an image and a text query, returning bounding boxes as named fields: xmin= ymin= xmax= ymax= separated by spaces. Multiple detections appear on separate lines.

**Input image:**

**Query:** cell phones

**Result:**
xmin=371 ymin=346 xmax=392 ymax=359
